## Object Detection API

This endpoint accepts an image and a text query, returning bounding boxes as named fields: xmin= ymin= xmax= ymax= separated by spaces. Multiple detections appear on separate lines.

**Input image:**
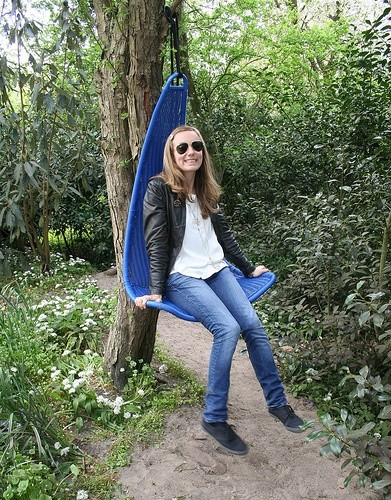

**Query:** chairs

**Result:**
xmin=120 ymin=71 xmax=276 ymax=323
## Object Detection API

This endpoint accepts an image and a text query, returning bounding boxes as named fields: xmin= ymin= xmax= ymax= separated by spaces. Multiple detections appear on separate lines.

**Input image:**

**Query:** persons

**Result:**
xmin=132 ymin=124 xmax=308 ymax=454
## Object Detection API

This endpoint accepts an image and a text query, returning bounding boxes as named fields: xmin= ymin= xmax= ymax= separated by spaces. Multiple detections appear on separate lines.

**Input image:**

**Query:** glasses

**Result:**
xmin=174 ymin=141 xmax=205 ymax=156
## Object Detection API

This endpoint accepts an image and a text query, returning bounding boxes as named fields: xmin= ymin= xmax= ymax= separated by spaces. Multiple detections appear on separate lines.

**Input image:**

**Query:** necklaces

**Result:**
xmin=185 ymin=194 xmax=202 ymax=228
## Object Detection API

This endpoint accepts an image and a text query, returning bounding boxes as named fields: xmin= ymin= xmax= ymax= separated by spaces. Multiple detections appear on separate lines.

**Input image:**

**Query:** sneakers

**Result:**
xmin=267 ymin=402 xmax=306 ymax=432
xmin=202 ymin=417 xmax=249 ymax=454
